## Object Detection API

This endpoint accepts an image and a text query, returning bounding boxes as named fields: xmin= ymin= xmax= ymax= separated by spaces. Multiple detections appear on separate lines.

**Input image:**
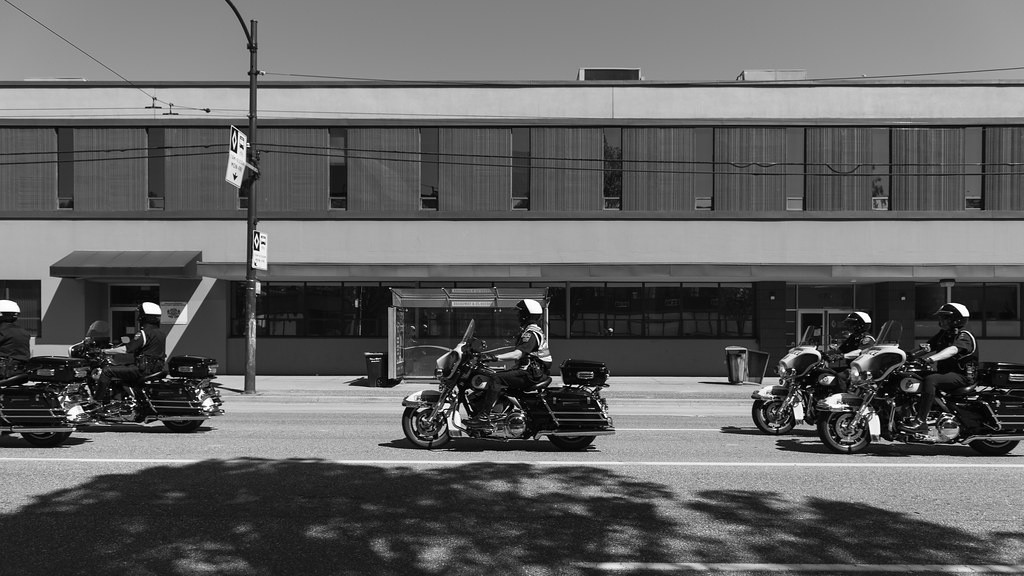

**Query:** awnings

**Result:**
xmin=49 ymin=251 xmax=202 ymax=278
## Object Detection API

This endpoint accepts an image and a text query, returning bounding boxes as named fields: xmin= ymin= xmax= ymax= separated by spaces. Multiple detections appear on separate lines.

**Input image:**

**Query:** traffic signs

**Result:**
xmin=251 ymin=230 xmax=268 ymax=272
xmin=225 ymin=125 xmax=248 ymax=189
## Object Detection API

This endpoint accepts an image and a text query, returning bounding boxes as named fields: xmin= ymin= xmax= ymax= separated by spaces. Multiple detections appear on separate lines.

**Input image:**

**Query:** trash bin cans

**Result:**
xmin=365 ymin=353 xmax=393 ymax=387
xmin=726 ymin=346 xmax=748 ymax=383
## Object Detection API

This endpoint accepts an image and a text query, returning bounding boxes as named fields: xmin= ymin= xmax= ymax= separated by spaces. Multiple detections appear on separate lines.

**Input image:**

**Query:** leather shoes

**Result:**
xmin=898 ymin=416 xmax=928 ymax=435
xmin=461 ymin=411 xmax=490 ymax=427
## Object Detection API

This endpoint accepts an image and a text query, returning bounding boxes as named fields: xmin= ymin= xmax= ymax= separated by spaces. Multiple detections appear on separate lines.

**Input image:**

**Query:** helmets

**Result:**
xmin=510 ymin=299 xmax=543 ymax=324
xmin=0 ymin=300 xmax=21 ymax=322
xmin=933 ymin=303 xmax=970 ymax=330
xmin=841 ymin=311 xmax=872 ymax=333
xmin=137 ymin=302 xmax=162 ymax=325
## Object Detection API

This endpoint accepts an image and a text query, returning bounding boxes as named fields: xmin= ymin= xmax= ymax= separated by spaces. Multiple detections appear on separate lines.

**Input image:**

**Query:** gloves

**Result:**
xmin=824 ymin=352 xmax=844 ymax=363
xmin=914 ymin=358 xmax=934 ymax=369
xmin=481 ymin=353 xmax=498 ymax=362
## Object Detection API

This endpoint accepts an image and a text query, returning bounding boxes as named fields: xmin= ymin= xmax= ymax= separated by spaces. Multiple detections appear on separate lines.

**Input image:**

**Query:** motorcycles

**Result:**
xmin=812 ymin=320 xmax=1024 ymax=456
xmin=69 ymin=320 xmax=226 ymax=433
xmin=750 ymin=325 xmax=849 ymax=436
xmin=0 ymin=357 xmax=92 ymax=448
xmin=402 ymin=318 xmax=616 ymax=452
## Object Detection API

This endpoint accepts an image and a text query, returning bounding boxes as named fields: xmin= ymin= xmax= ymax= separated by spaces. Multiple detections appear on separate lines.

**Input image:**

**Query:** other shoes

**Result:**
xmin=82 ymin=397 xmax=112 ymax=411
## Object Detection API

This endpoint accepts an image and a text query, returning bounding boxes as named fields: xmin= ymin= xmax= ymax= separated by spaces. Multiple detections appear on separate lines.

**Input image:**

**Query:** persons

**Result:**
xmin=461 ymin=299 xmax=553 ymax=427
xmin=78 ymin=302 xmax=166 ymax=398
xmin=0 ymin=299 xmax=31 ymax=381
xmin=898 ymin=303 xmax=979 ymax=435
xmin=811 ymin=311 xmax=876 ymax=417
